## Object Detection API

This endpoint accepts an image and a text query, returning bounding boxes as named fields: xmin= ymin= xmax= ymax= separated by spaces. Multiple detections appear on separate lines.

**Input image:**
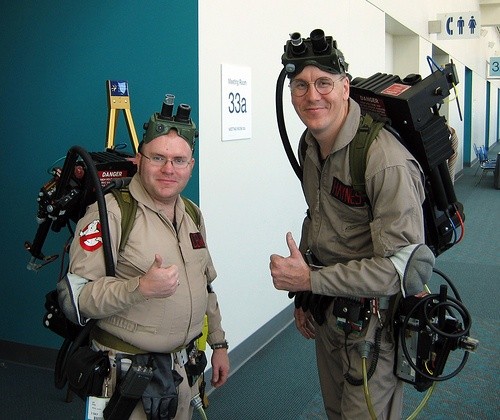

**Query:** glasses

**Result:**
xmin=139 ymin=152 xmax=191 ymax=169
xmin=288 ymin=76 xmax=344 ymax=97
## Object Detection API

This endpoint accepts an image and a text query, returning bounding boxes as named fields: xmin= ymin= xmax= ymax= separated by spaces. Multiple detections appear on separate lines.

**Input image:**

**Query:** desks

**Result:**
xmin=493 ymin=152 xmax=500 ymax=189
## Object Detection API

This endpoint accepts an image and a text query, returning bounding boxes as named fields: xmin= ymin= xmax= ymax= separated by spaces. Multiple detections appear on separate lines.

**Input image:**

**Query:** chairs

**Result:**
xmin=473 ymin=144 xmax=496 ymax=187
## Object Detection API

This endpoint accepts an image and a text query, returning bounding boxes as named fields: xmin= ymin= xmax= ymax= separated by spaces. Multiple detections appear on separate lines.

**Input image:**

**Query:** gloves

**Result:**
xmin=287 ymin=289 xmax=332 ymax=325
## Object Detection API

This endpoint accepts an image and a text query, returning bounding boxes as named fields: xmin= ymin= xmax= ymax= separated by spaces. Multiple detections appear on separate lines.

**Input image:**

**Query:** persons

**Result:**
xmin=269 ymin=28 xmax=434 ymax=420
xmin=55 ymin=95 xmax=231 ymax=420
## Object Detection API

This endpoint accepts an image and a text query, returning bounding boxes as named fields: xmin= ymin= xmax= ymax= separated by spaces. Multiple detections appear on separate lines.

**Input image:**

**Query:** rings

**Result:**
xmin=176 ymin=280 xmax=180 ymax=285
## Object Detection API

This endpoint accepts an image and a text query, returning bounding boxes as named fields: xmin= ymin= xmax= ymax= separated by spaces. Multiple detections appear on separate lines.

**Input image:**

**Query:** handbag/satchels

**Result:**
xmin=65 ymin=347 xmax=109 ymax=401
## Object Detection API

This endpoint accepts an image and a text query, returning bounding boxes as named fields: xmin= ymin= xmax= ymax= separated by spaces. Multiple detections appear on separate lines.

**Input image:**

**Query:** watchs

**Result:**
xmin=211 ymin=340 xmax=229 ymax=350
